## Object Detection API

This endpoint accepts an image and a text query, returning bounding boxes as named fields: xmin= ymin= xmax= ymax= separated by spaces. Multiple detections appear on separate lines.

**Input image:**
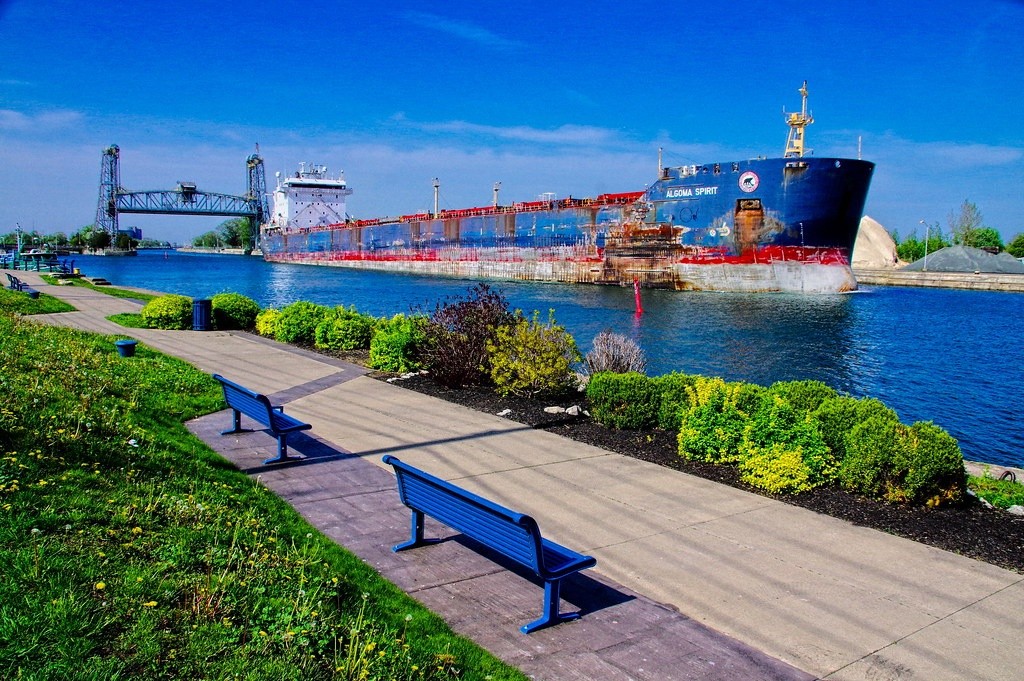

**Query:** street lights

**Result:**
xmin=920 ymin=220 xmax=929 ymax=271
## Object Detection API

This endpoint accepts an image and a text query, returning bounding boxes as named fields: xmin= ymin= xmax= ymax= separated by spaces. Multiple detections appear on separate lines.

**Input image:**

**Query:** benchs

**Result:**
xmin=13 ymin=276 xmax=29 ymax=291
xmin=211 ymin=373 xmax=312 ymax=466
xmin=4 ymin=272 xmax=14 ymax=289
xmin=381 ymin=454 xmax=597 ymax=633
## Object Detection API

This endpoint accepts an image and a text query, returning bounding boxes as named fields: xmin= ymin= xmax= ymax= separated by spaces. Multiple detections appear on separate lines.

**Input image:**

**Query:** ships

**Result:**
xmin=255 ymin=79 xmax=876 ymax=294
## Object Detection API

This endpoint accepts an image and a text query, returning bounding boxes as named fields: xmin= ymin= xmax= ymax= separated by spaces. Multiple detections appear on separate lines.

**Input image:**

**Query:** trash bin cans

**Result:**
xmin=193 ymin=299 xmax=212 ymax=331
